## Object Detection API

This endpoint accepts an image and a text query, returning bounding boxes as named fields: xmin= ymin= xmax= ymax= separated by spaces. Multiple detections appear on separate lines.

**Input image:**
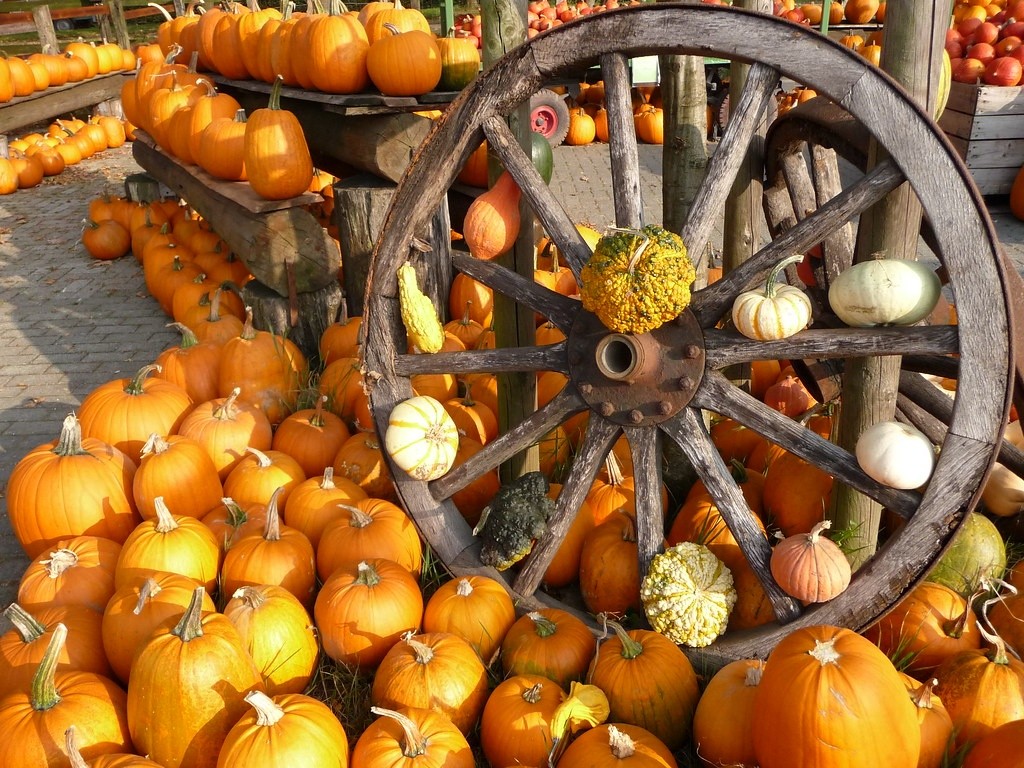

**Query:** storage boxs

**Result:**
xmin=935 ymin=81 xmax=1024 ymax=195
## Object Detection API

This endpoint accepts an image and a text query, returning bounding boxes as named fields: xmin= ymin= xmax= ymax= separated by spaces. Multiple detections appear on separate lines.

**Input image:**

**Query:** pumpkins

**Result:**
xmin=1 ymin=0 xmax=1024 ymax=768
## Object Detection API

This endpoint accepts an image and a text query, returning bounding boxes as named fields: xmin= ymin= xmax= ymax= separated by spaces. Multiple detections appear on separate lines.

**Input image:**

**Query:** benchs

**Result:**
xmin=0 ymin=66 xmax=133 ymax=162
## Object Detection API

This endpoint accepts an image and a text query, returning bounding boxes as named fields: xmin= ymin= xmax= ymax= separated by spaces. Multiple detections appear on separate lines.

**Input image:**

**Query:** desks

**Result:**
xmin=124 ymin=74 xmax=461 ymax=373
